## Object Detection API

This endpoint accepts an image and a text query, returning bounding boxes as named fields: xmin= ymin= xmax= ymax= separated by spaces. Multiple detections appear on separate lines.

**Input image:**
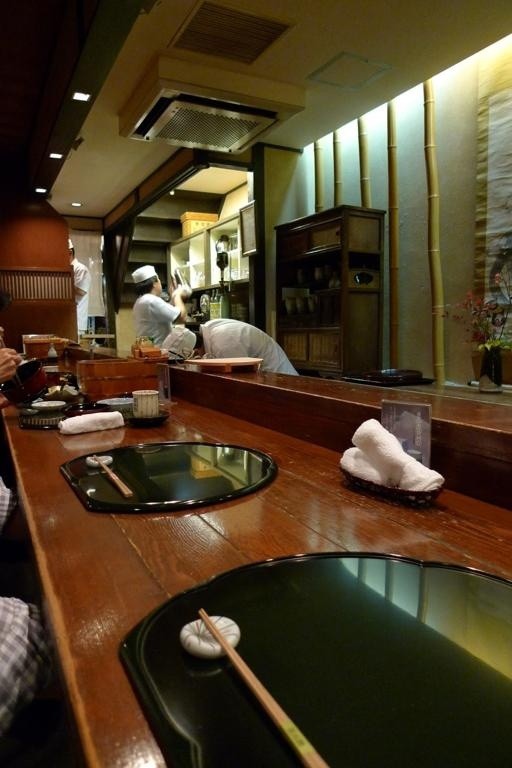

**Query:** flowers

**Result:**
xmin=440 ymin=270 xmax=512 ymax=350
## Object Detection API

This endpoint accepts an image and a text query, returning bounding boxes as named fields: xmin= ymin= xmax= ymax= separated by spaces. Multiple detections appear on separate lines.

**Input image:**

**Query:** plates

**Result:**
xmin=17 ymin=365 xmax=172 ymax=431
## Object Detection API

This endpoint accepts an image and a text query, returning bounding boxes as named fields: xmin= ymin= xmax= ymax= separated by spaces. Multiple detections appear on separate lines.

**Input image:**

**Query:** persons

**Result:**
xmin=163 ymin=318 xmax=300 ymax=376
xmin=132 ymin=265 xmax=186 ymax=347
xmin=1 ymin=348 xmax=22 ymax=383
xmin=69 ymin=240 xmax=90 ymax=340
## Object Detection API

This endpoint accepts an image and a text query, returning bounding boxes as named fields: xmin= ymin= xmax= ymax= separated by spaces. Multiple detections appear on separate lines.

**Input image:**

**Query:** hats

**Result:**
xmin=131 ymin=265 xmax=156 ymax=284
xmin=163 ymin=324 xmax=197 ymax=355
xmin=68 ymin=238 xmax=74 ymax=250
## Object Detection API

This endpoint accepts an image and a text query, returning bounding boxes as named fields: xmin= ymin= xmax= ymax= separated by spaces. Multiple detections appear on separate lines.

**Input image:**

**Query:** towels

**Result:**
xmin=351 ymin=416 xmax=451 ymax=499
xmin=57 ymin=406 xmax=128 ymax=438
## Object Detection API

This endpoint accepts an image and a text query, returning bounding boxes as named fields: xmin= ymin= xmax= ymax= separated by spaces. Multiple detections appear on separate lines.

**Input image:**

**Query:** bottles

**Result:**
xmin=281 ymin=268 xmax=343 ymax=320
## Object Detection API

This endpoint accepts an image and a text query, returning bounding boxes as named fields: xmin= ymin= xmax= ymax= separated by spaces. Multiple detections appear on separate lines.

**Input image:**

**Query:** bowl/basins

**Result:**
xmin=1 ymin=360 xmax=51 ymax=409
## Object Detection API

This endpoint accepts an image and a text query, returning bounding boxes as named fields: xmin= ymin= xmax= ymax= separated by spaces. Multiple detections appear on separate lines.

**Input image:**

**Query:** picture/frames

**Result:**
xmin=239 ymin=200 xmax=261 ymax=256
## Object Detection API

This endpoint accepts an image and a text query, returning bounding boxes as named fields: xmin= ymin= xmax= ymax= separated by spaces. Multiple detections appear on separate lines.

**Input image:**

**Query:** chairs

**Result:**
xmin=343 ymin=362 xmax=426 ymax=393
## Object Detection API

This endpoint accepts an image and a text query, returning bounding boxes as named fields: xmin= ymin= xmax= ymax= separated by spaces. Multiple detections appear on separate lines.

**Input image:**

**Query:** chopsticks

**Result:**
xmin=91 ymin=452 xmax=136 ymax=499
xmin=195 ymin=607 xmax=329 ymax=768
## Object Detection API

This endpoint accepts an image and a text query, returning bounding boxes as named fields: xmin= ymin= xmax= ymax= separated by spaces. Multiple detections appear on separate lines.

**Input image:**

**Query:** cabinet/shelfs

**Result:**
xmin=207 ymin=208 xmax=249 ymax=289
xmin=273 ymin=204 xmax=386 ymax=379
xmin=166 ymin=229 xmax=208 ymax=294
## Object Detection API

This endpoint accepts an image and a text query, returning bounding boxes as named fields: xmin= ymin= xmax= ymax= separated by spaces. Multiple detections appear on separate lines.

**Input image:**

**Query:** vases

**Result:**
xmin=479 ymin=346 xmax=506 ymax=394
